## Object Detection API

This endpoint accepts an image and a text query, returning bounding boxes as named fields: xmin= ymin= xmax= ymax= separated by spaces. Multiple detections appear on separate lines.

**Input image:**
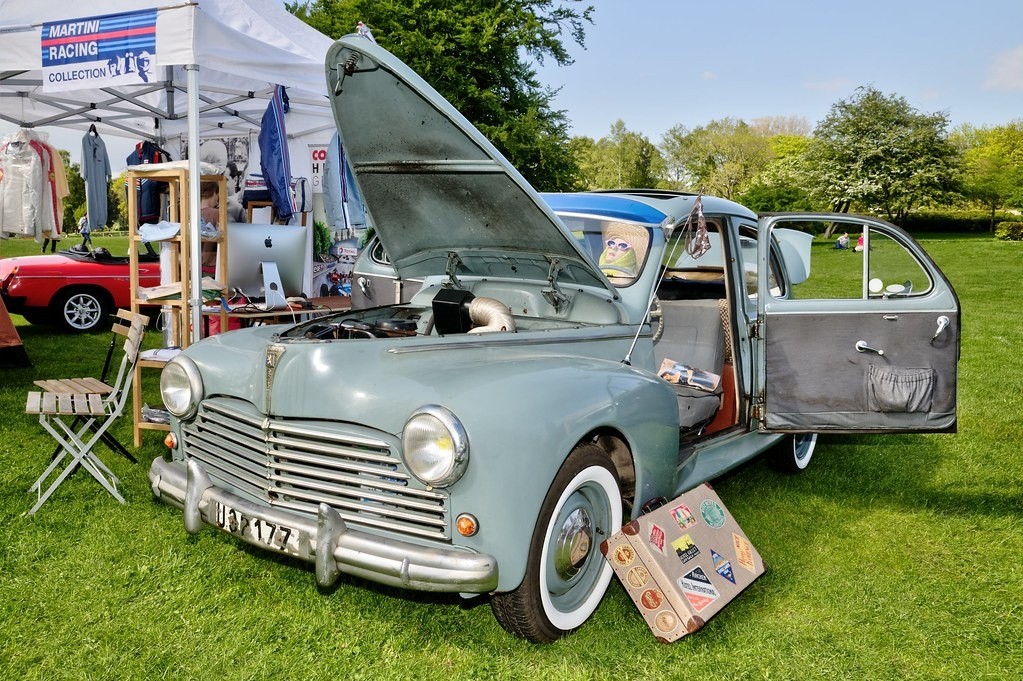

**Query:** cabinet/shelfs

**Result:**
xmin=128 ymin=170 xmax=229 ymax=446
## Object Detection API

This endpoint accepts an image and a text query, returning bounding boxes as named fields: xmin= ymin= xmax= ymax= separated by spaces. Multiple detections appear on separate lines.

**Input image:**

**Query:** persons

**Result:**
xmin=42 ymin=238 xmax=56 ymax=253
xmin=188 ymin=182 xmax=219 ymax=280
xmin=78 ymin=212 xmax=95 ymax=252
xmin=661 ymin=371 xmax=680 ymax=383
xmin=835 ymin=231 xmax=850 ymax=251
xmin=855 ymin=233 xmax=863 ymax=252
xmin=599 ymin=220 xmax=649 ymax=285
xmin=223 ymin=138 xmax=248 ymax=203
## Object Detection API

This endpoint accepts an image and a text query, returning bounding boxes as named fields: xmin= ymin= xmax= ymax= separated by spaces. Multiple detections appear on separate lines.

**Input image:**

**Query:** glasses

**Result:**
xmin=607 ymin=241 xmax=633 ymax=251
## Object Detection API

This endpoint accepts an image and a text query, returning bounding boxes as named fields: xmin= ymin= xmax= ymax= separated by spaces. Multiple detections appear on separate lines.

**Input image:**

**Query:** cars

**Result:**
xmin=148 ymin=20 xmax=967 ymax=656
xmin=0 ymin=231 xmax=209 ymax=337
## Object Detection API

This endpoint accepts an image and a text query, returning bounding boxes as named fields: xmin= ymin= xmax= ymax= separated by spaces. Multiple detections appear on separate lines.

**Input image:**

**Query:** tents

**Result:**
xmin=0 ymin=0 xmax=337 ymax=342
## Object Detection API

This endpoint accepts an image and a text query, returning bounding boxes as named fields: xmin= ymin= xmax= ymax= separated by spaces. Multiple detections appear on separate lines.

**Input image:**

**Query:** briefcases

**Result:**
xmin=601 ymin=480 xmax=766 ymax=645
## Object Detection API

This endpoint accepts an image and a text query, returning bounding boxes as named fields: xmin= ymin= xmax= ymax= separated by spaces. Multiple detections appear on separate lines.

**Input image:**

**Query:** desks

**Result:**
xmin=202 ymin=297 xmax=353 ymax=317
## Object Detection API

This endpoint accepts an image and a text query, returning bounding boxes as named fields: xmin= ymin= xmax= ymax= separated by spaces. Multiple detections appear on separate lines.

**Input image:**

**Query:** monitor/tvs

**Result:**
xmin=215 ymin=222 xmax=307 ymax=311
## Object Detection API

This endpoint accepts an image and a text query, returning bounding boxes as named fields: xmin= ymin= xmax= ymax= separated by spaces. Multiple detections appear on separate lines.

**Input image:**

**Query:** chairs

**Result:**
xmin=651 ymin=300 xmax=724 ymax=421
xmin=69 ymin=244 xmax=112 ymax=258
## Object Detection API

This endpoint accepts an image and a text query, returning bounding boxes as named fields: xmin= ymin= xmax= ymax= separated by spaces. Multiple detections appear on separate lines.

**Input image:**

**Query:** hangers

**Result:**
xmin=8 ymin=122 xmax=165 ymax=150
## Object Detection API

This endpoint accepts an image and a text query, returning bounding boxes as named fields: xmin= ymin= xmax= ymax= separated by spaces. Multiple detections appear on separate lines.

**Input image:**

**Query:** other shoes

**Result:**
xmin=51 ymin=249 xmax=55 ymax=253
xmin=42 ymin=246 xmax=45 ymax=254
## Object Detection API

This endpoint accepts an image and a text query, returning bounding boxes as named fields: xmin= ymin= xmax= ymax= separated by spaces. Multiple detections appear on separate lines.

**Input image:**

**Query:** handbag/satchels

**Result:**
xmin=78 ymin=225 xmax=82 ymax=229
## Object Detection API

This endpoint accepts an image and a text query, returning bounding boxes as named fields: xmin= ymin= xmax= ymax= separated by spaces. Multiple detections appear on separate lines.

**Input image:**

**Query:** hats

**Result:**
xmin=605 ymin=220 xmax=649 ymax=270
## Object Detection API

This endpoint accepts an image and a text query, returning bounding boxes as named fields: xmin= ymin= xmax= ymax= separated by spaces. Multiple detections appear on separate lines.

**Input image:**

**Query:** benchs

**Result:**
xmin=28 ymin=308 xmax=151 ymax=515
